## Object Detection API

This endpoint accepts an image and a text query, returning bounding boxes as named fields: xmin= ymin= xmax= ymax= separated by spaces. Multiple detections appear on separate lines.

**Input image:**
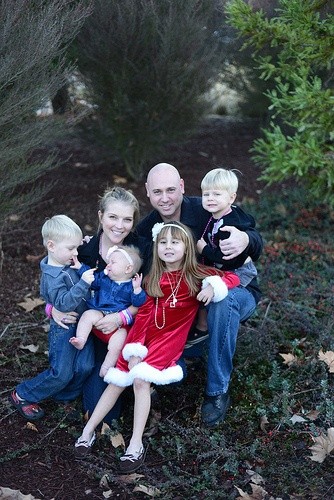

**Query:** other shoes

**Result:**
xmin=201 ymin=392 xmax=230 ymax=427
xmin=186 ymin=327 xmax=209 ymax=345
xmin=72 ymin=429 xmax=96 ymax=458
xmin=118 ymin=443 xmax=147 ymax=472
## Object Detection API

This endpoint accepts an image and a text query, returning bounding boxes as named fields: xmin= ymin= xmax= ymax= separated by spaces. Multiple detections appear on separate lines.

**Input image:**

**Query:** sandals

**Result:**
xmin=10 ymin=388 xmax=44 ymax=420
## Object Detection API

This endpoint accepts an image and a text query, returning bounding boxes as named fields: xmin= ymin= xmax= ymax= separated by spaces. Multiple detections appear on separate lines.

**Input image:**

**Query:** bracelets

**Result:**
xmin=45 ymin=304 xmax=51 ymax=318
xmin=120 ymin=308 xmax=133 ymax=326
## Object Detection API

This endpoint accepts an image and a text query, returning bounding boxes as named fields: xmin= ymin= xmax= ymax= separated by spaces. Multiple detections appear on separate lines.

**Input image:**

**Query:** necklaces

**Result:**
xmin=155 ymin=271 xmax=184 ymax=330
xmin=165 ymin=267 xmax=183 ymax=304
xmin=201 ymin=208 xmax=230 ymax=267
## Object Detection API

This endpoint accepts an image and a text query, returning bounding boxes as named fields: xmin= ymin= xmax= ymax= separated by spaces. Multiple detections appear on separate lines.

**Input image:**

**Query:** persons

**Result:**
xmin=56 ymin=187 xmax=153 ymax=426
xmin=68 ymin=244 xmax=147 ymax=379
xmin=10 ymin=215 xmax=94 ymax=421
xmin=127 ymin=162 xmax=264 ymax=426
xmin=183 ymin=167 xmax=253 ymax=349
xmin=72 ymin=220 xmax=240 ymax=474
